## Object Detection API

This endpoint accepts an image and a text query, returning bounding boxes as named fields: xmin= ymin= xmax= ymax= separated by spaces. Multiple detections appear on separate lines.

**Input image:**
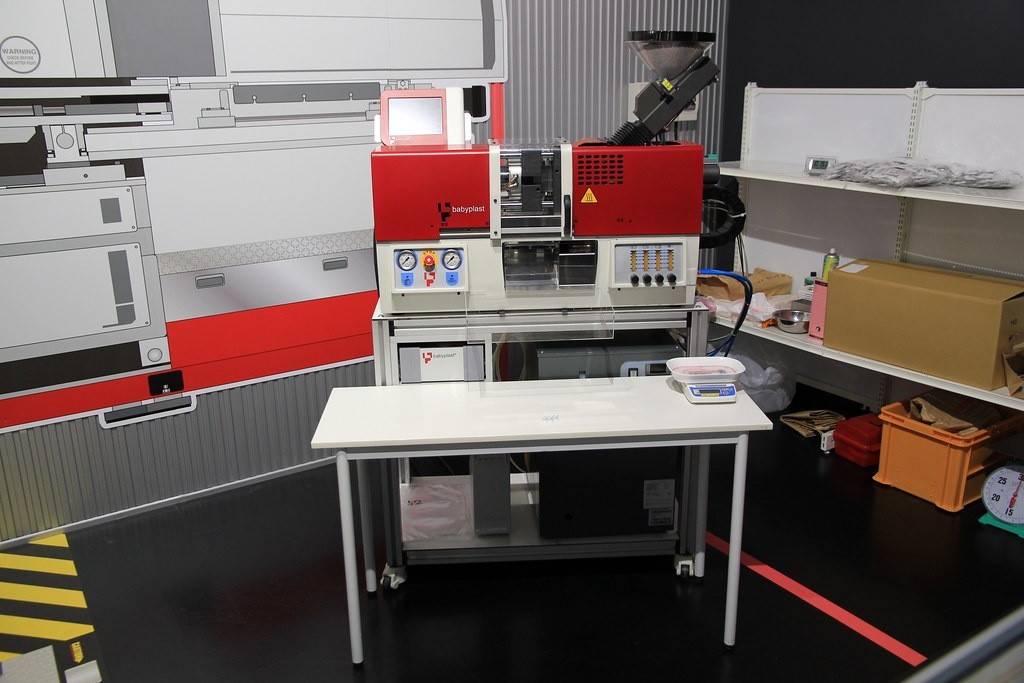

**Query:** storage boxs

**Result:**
xmin=791 ymin=278 xmax=829 ymax=340
xmin=833 ymin=413 xmax=881 ymax=466
xmin=871 ymin=391 xmax=1024 ymax=512
xmin=822 ymin=260 xmax=1023 ymax=390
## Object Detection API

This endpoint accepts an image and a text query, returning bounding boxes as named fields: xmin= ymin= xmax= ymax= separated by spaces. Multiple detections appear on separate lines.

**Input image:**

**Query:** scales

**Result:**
xmin=804 ymin=155 xmax=836 ymax=175
xmin=977 ymin=433 xmax=1024 ymax=539
xmin=665 ymin=357 xmax=746 ymax=404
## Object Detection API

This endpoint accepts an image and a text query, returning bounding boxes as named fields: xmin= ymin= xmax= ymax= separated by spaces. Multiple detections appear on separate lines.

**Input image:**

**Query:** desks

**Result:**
xmin=311 ymin=374 xmax=772 ymax=669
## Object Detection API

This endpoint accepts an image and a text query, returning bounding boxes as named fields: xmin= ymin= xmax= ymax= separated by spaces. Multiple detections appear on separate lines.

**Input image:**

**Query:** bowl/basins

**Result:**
xmin=772 ymin=309 xmax=811 ymax=333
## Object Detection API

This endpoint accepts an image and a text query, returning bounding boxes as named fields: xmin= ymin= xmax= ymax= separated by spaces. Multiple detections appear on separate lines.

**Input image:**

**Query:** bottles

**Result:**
xmin=821 ymin=248 xmax=840 ymax=282
xmin=803 ymin=272 xmax=820 ymax=301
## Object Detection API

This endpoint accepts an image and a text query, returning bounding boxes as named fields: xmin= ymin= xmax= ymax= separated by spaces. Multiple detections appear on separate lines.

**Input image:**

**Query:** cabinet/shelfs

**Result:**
xmin=710 ymin=81 xmax=1023 ymax=413
